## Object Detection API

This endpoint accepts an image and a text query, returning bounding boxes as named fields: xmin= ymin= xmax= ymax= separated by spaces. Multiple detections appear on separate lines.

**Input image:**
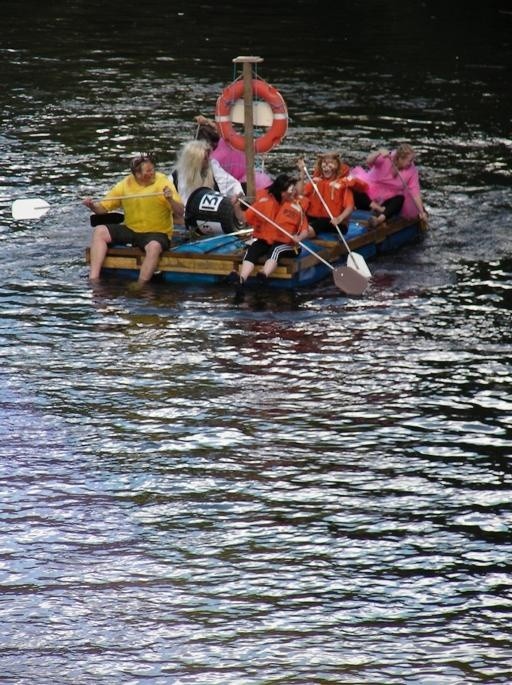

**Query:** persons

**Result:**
xmin=191 ymin=115 xmax=274 ymax=212
xmin=348 ymin=143 xmax=427 ymax=227
xmin=294 ymin=151 xmax=355 ymax=238
xmin=82 ymin=155 xmax=184 ymax=283
xmin=231 ymin=174 xmax=309 ymax=291
xmin=167 ymin=140 xmax=245 ymax=217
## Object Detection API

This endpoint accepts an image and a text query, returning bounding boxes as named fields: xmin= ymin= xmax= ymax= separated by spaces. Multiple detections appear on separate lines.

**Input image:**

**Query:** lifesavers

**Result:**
xmin=215 ymin=80 xmax=288 ymax=152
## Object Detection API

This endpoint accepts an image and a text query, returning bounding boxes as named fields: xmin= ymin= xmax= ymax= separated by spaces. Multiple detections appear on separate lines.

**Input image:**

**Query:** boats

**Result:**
xmin=77 ymin=56 xmax=433 ymax=290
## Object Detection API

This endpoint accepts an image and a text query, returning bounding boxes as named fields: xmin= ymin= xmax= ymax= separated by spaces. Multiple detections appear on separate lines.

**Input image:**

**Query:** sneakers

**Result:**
xmin=225 ymin=268 xmax=245 ymax=292
xmin=367 ymin=215 xmax=379 ymax=230
xmin=253 ymin=270 xmax=268 ymax=291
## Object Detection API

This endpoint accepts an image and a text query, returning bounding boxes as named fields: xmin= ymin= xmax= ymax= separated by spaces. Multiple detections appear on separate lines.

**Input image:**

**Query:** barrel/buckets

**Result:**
xmin=184 ymin=187 xmax=251 ymax=234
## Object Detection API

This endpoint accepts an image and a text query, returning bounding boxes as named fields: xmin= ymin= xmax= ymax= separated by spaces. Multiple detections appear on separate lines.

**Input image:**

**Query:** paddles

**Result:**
xmin=237 ymin=197 xmax=368 ymax=295
xmin=12 ymin=192 xmax=164 ymax=220
xmin=303 ymin=165 xmax=372 ymax=280
